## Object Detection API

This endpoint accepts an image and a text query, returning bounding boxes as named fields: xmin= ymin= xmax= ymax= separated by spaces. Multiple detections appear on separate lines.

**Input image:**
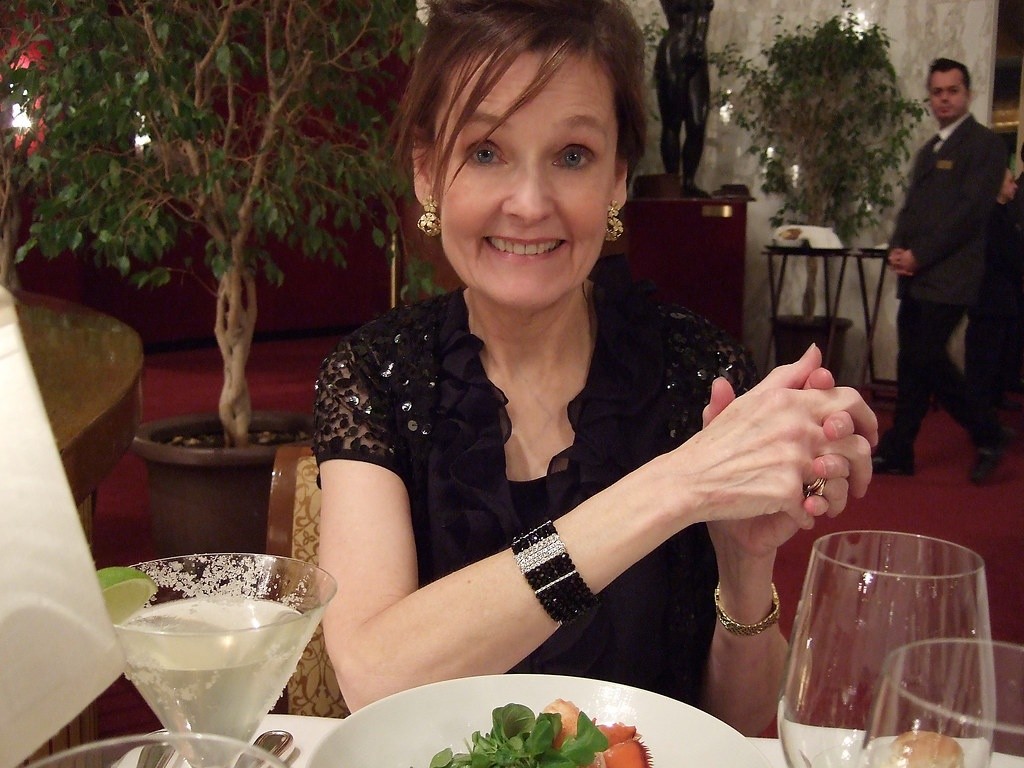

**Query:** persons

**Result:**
xmin=966 ymin=140 xmax=1024 ymax=414
xmin=307 ymin=0 xmax=880 ymax=740
xmin=653 ymin=0 xmax=715 ymax=201
xmin=867 ymin=56 xmax=1014 ymax=482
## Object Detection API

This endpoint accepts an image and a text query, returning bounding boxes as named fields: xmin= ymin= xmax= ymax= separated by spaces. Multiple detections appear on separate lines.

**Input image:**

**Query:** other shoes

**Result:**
xmin=971 ymin=428 xmax=1013 ymax=479
xmin=872 ymin=456 xmax=914 ymax=476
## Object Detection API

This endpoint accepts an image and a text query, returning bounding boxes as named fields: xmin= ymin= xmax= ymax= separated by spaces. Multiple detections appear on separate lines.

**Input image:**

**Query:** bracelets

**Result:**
xmin=510 ymin=517 xmax=602 ymax=629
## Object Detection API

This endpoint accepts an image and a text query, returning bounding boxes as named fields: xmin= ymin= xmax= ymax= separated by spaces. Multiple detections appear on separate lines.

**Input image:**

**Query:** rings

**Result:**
xmin=803 ymin=476 xmax=827 ymax=500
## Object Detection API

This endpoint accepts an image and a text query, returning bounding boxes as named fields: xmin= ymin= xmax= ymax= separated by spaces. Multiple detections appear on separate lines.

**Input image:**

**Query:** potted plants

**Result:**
xmin=709 ymin=18 xmax=925 ymax=387
xmin=0 ymin=0 xmax=424 ymax=559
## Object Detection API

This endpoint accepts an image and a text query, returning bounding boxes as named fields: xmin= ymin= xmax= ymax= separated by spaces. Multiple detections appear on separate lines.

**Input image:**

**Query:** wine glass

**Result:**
xmin=110 ymin=551 xmax=337 ymax=768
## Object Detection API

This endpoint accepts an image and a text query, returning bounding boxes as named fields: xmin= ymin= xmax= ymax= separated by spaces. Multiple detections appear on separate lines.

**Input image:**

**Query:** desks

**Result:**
xmin=760 ymin=243 xmax=898 ymax=410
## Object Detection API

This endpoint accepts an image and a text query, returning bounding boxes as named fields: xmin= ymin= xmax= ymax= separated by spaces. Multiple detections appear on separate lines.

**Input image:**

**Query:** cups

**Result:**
xmin=23 ymin=729 xmax=283 ymax=768
xmin=776 ymin=532 xmax=994 ymax=768
xmin=862 ymin=637 xmax=1024 ymax=768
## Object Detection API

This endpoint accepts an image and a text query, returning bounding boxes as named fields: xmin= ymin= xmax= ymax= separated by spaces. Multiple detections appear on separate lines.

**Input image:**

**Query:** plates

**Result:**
xmin=302 ymin=673 xmax=774 ymax=768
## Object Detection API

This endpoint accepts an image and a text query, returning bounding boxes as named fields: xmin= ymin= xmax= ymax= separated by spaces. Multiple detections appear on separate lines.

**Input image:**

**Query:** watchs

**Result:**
xmin=711 ymin=582 xmax=781 ymax=638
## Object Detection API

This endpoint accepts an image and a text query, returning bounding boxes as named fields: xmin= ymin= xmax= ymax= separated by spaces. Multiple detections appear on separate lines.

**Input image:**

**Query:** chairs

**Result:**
xmin=258 ymin=446 xmax=354 ymax=719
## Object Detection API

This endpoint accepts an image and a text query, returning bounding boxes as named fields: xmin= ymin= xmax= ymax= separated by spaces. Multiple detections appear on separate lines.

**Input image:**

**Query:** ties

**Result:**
xmin=923 ymin=134 xmax=941 ymax=157
